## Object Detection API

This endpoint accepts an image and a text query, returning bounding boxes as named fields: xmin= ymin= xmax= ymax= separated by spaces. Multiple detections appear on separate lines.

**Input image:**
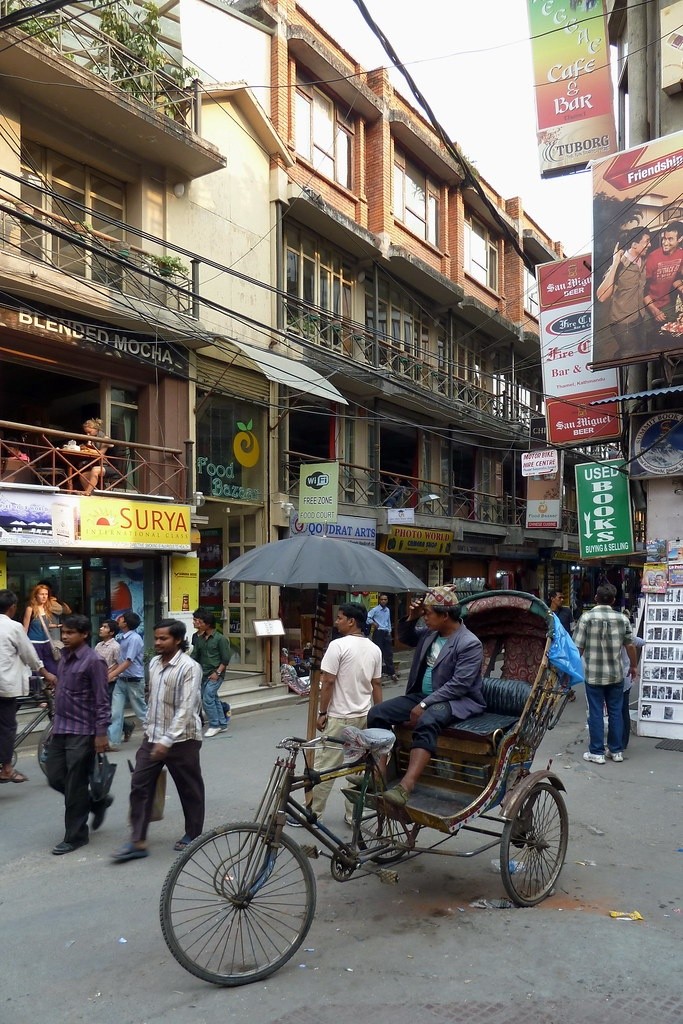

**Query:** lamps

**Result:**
xmin=419 ymin=494 xmax=449 ymax=518
xmin=672 ymin=480 xmax=683 ymax=495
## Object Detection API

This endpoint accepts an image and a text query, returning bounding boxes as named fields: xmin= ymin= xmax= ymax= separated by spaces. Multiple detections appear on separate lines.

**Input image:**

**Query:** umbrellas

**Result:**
xmin=211 ymin=534 xmax=432 ymax=593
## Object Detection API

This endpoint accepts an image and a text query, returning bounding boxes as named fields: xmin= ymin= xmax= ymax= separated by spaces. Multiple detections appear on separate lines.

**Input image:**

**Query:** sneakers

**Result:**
xmin=286 ymin=814 xmax=323 ymax=828
xmin=583 ymin=752 xmax=606 ymax=764
xmin=383 ymin=783 xmax=411 ymax=808
xmin=605 ymin=749 xmax=624 ymax=761
xmin=346 ymin=774 xmax=388 ymax=792
xmin=343 ymin=812 xmax=364 ymax=830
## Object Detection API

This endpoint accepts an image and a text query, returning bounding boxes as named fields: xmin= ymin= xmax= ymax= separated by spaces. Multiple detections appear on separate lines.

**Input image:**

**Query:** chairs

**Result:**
xmin=36 ymin=450 xmax=70 ymax=491
xmin=104 ymin=447 xmax=132 ymax=492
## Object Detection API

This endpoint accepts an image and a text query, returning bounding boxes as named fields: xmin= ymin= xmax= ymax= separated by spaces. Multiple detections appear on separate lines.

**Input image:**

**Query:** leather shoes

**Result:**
xmin=52 ymin=837 xmax=89 ymax=855
xmin=92 ymin=796 xmax=113 ymax=830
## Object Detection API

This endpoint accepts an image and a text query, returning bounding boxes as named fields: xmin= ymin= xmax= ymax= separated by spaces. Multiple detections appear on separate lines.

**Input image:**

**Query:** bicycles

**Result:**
xmin=0 ymin=676 xmax=57 ymax=776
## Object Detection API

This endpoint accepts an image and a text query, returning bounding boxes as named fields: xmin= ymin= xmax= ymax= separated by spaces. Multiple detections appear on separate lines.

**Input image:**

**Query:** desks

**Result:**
xmin=36 ymin=450 xmax=100 ymax=493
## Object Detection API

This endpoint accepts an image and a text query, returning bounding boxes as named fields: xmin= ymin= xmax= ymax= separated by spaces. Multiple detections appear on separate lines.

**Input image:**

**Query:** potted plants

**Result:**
xmin=152 ymin=256 xmax=189 ymax=276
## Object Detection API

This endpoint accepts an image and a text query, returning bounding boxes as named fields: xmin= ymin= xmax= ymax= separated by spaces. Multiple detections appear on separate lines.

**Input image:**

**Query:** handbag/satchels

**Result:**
xmin=50 ymin=639 xmax=64 ymax=661
xmin=87 ymin=749 xmax=117 ymax=802
xmin=128 ymin=765 xmax=167 ymax=824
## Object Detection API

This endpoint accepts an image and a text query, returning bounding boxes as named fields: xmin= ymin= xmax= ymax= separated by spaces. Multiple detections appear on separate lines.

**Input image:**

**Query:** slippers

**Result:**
xmin=0 ymin=770 xmax=31 ymax=783
xmin=109 ymin=843 xmax=147 ymax=861
xmin=175 ymin=834 xmax=197 ymax=851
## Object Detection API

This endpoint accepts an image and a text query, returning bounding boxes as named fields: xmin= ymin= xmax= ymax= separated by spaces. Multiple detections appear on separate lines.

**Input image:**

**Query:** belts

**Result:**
xmin=119 ymin=677 xmax=143 ymax=682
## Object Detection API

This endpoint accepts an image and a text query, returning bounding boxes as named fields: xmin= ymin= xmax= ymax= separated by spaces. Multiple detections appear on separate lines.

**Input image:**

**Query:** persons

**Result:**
xmin=6 ymin=436 xmax=30 ymax=461
xmin=642 ymin=221 xmax=683 ymax=348
xmin=645 ymin=569 xmax=665 ymax=587
xmin=96 ymin=612 xmax=149 ymax=753
xmin=619 ymin=635 xmax=646 ymax=749
xmin=284 ymin=601 xmax=382 ymax=828
xmin=572 ymin=582 xmax=638 ymax=764
xmin=78 ymin=418 xmax=119 ymax=496
xmin=366 ymin=593 xmax=399 ymax=681
xmin=0 ymin=589 xmax=58 ymax=784
xmin=197 ymin=612 xmax=228 ymax=737
xmin=23 ymin=584 xmax=58 ymax=708
xmin=595 ymin=226 xmax=652 ymax=357
xmin=95 ymin=619 xmax=135 ymax=742
xmin=46 ymin=614 xmax=114 ymax=856
xmin=547 ymin=589 xmax=577 ymax=702
xmin=345 ymin=584 xmax=488 ymax=808
xmin=110 ymin=618 xmax=205 ymax=860
xmin=192 ymin=607 xmax=231 ymax=727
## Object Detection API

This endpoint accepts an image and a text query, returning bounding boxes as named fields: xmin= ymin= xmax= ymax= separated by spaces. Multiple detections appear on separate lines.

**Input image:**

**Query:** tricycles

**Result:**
xmin=159 ymin=589 xmax=586 ymax=987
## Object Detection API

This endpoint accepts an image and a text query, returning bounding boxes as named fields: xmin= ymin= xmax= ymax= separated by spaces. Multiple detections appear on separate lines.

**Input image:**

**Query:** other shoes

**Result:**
xmin=217 ymin=723 xmax=229 ymax=732
xmin=204 ymin=728 xmax=221 ymax=736
xmin=391 ymin=674 xmax=398 ymax=681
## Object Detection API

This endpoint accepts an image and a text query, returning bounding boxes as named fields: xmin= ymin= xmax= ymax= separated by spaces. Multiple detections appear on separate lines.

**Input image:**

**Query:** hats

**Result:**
xmin=423 ymin=584 xmax=459 ymax=605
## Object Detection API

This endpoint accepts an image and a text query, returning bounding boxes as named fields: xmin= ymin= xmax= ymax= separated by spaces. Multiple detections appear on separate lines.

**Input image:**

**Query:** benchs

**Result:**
xmin=393 ymin=678 xmax=533 ymax=756
